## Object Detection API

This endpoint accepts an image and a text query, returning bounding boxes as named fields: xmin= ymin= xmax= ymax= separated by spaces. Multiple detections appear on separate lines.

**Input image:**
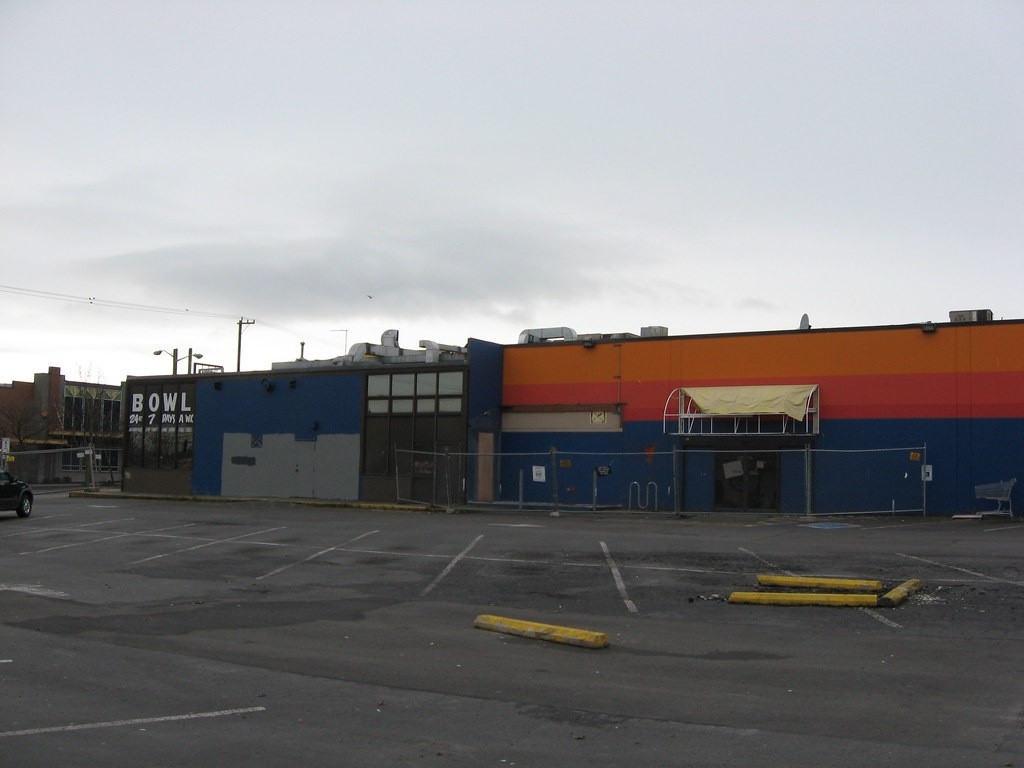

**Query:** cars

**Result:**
xmin=0 ymin=467 xmax=35 ymax=520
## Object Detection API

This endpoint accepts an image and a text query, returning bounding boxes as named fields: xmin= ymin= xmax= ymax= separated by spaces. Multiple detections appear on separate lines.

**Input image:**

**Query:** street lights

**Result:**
xmin=153 ymin=349 xmax=205 ymax=375
